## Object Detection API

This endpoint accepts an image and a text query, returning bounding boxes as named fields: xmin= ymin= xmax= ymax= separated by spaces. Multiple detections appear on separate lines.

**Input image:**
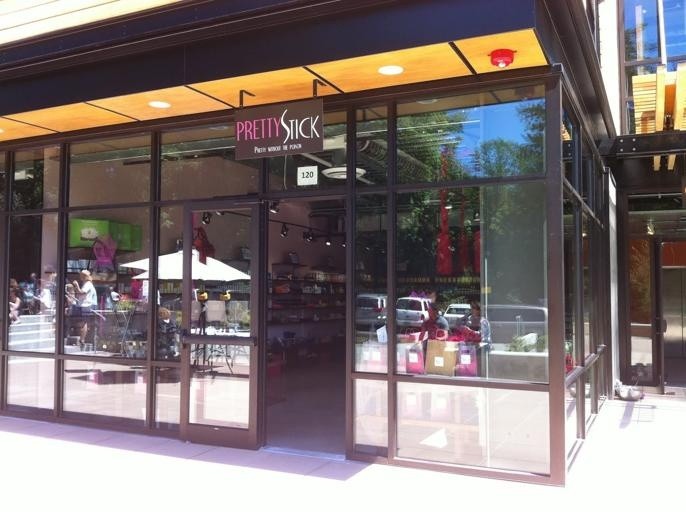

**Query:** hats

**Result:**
xmin=81 ymin=269 xmax=92 ymax=281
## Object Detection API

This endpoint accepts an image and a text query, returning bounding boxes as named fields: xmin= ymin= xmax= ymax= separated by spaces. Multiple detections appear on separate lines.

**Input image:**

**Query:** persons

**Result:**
xmin=63 ymin=283 xmax=76 ymax=306
xmin=73 ymin=270 xmax=98 ymax=352
xmin=419 ymin=302 xmax=449 ymax=367
xmin=456 ymin=302 xmax=491 ymax=376
xmin=8 ymin=273 xmax=52 ymax=324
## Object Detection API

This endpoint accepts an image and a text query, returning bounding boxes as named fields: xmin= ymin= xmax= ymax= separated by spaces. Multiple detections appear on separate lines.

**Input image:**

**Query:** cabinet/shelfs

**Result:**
xmin=264 ymin=276 xmax=481 ymax=342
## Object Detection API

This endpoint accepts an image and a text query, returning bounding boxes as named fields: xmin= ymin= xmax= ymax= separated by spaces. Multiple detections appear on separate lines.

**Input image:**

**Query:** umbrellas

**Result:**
xmin=119 ymin=247 xmax=252 ymax=365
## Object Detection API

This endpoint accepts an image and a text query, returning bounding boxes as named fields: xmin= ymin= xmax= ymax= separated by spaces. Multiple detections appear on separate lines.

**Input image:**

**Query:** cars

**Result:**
xmin=354 ymin=294 xmax=388 ymax=329
xmin=443 ymin=303 xmax=472 ymax=330
xmin=395 ymin=297 xmax=433 ymax=332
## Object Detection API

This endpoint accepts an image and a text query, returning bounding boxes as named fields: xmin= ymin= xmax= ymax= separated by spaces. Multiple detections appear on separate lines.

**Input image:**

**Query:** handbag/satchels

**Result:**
xmin=454 ymin=344 xmax=477 ymax=376
xmin=363 ymin=338 xmax=424 ymax=374
xmin=425 ymin=338 xmax=457 ymax=376
xmin=399 ymin=328 xmax=429 ymax=343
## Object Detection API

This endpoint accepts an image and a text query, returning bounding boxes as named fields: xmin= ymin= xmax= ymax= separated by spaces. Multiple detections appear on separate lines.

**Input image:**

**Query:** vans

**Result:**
xmin=484 ymin=304 xmax=548 ymax=345
